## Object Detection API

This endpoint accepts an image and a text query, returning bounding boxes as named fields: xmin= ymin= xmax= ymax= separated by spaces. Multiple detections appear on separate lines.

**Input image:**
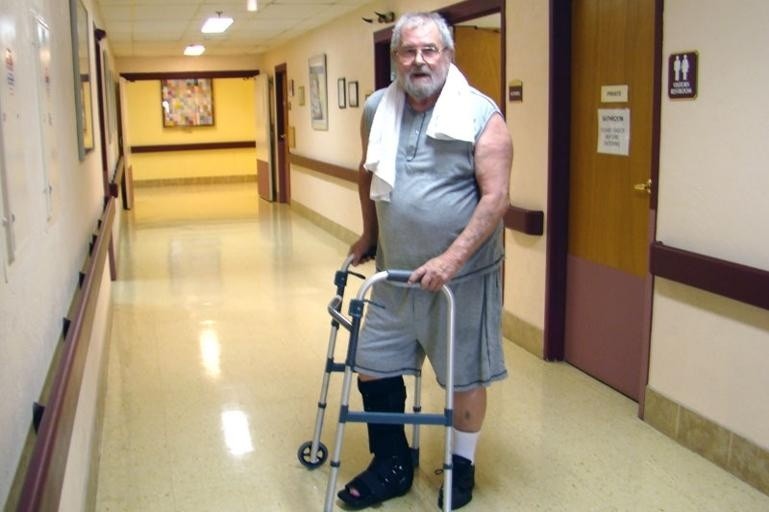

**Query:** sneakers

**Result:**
xmin=438 ymin=455 xmax=475 ymax=510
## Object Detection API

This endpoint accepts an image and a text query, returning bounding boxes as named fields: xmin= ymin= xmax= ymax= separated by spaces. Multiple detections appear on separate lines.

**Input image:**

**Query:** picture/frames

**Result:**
xmin=336 ymin=77 xmax=359 ymax=109
xmin=160 ymin=77 xmax=215 ymax=129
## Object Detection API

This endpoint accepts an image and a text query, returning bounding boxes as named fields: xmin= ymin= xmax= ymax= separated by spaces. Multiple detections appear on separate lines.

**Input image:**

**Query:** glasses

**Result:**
xmin=396 ymin=47 xmax=448 ymax=60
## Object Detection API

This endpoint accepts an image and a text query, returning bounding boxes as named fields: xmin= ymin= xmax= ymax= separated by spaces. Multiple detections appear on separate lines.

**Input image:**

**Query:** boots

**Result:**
xmin=336 ymin=374 xmax=414 ymax=510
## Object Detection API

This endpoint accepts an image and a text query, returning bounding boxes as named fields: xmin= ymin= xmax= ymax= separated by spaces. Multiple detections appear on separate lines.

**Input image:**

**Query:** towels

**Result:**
xmin=363 ymin=63 xmax=477 ymax=204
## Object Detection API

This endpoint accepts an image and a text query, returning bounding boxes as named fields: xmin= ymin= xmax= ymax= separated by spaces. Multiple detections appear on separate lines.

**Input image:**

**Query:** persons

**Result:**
xmin=337 ymin=10 xmax=514 ymax=510
